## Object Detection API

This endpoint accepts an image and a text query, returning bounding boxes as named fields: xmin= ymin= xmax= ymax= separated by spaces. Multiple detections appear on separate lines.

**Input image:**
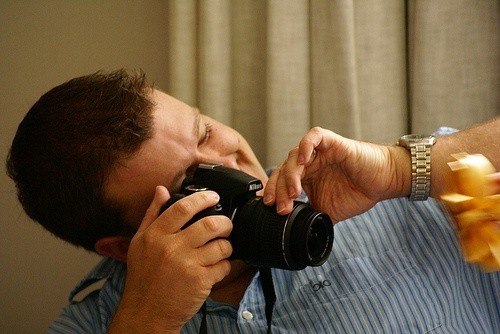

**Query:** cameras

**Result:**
xmin=158 ymin=160 xmax=336 ymax=274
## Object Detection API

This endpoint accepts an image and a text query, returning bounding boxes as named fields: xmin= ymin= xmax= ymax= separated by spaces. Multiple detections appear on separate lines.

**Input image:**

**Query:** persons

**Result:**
xmin=7 ymin=66 xmax=500 ymax=333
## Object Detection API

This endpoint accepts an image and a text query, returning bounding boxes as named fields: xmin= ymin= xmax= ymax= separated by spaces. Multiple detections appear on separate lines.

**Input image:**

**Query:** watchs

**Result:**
xmin=395 ymin=132 xmax=437 ymax=201
